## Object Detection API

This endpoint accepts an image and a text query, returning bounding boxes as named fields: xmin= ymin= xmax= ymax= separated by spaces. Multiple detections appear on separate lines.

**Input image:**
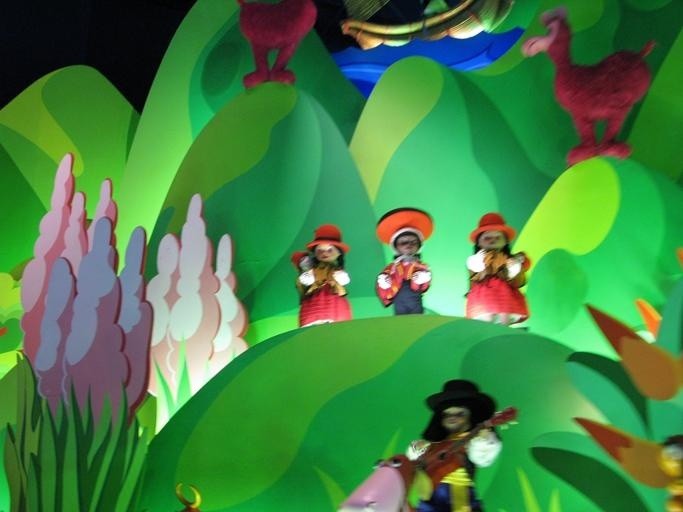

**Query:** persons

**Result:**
xmin=289 ymin=223 xmax=358 ymax=329
xmin=403 ymin=379 xmax=501 ymax=511
xmin=374 ymin=207 xmax=434 ymax=314
xmin=464 ymin=213 xmax=530 ymax=326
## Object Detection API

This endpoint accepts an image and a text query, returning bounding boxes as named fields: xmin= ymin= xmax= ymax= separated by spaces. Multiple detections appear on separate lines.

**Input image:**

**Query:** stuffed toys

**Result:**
xmin=293 ymin=250 xmax=315 ymax=276
xmin=237 ymin=0 xmax=317 ymax=89
xmin=521 ymin=8 xmax=658 ymax=167
xmin=498 ymin=250 xmax=531 ymax=282
